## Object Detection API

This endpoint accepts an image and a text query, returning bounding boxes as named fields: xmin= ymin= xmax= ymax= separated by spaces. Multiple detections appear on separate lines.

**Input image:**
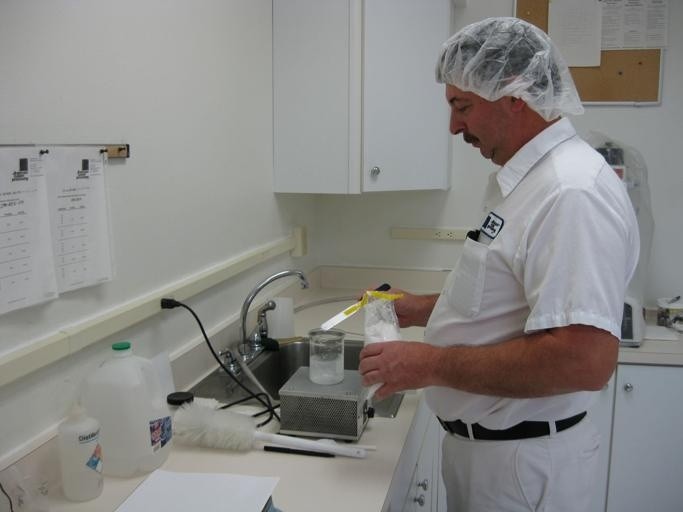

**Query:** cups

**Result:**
xmin=308 ymin=327 xmax=347 ymax=385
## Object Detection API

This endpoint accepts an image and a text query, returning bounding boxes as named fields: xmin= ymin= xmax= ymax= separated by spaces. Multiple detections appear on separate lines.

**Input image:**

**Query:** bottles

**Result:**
xmin=57 ymin=401 xmax=105 ymax=502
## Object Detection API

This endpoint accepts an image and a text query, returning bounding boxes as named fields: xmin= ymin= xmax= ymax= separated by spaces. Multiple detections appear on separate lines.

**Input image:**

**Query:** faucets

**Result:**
xmin=239 ymin=269 xmax=310 ymax=360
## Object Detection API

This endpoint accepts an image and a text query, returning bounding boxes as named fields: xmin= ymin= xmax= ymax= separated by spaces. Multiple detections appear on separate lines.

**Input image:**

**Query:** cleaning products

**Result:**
xmin=60 ymin=376 xmax=103 ymax=504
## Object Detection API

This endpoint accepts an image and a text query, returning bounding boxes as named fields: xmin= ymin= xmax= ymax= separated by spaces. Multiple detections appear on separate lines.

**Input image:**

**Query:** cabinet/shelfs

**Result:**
xmin=270 ymin=0 xmax=456 ymax=195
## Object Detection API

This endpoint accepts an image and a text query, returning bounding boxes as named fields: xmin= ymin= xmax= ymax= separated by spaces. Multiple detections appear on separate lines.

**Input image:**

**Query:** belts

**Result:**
xmin=436 ymin=407 xmax=587 ymax=441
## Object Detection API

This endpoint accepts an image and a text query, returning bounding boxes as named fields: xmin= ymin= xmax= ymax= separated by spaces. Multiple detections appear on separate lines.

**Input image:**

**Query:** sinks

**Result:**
xmin=186 ymin=337 xmax=405 ymax=419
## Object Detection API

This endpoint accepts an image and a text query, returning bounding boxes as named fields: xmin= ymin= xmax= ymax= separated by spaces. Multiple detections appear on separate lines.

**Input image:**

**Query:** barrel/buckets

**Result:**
xmin=79 ymin=342 xmax=172 ymax=477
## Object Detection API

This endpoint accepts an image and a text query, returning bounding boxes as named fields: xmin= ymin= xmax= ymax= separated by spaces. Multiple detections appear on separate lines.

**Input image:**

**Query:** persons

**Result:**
xmin=359 ymin=16 xmax=642 ymax=512
